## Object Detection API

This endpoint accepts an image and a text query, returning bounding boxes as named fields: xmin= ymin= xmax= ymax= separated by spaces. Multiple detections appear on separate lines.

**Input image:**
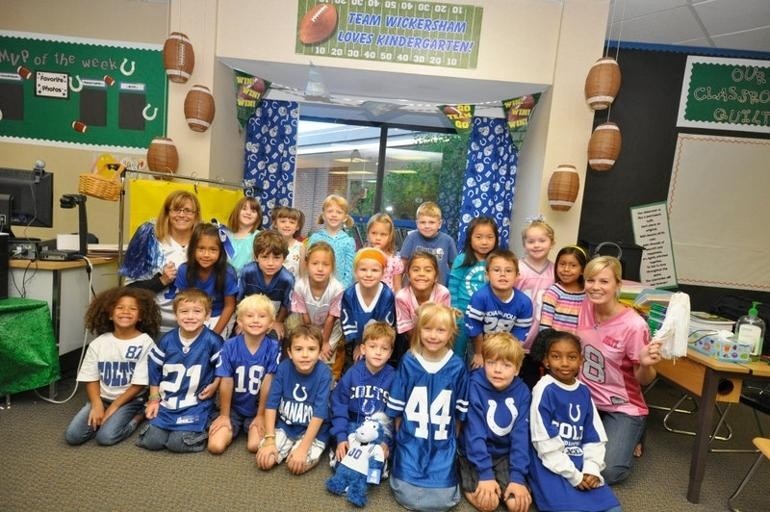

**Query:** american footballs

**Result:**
xmin=299 ymin=3 xmax=338 ymax=45
xmin=584 ymin=57 xmax=622 ymax=111
xmin=184 ymin=84 xmax=216 ymax=134
xmin=587 ymin=122 xmax=623 ymax=172
xmin=163 ymin=31 xmax=196 ymax=84
xmin=146 ymin=137 xmax=180 ymax=182
xmin=547 ymin=164 xmax=580 ymax=212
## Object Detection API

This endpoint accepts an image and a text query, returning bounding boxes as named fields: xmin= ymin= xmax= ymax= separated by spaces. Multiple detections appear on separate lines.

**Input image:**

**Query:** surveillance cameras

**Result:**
xmin=33 ymin=160 xmax=46 ymax=184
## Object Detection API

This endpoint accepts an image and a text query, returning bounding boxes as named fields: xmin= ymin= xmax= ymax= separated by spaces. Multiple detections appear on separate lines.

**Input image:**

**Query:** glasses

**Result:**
xmin=489 ymin=266 xmax=517 ymax=274
xmin=169 ymin=208 xmax=197 ymax=215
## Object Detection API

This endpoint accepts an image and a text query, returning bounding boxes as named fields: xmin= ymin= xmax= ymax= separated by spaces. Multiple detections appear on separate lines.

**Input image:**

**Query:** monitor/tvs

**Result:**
xmin=0 ymin=167 xmax=53 ymax=241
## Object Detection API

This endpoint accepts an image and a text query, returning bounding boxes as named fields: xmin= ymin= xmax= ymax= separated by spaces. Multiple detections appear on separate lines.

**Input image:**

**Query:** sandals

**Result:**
xmin=631 ymin=431 xmax=645 ymax=458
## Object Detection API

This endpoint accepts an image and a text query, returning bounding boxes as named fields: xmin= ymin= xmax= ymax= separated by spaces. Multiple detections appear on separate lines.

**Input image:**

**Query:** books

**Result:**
xmin=647 ymin=302 xmax=736 ymax=345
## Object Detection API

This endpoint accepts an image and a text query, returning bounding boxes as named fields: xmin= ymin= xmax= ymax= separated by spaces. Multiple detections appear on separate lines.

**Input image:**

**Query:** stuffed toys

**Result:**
xmin=324 ymin=412 xmax=393 ymax=508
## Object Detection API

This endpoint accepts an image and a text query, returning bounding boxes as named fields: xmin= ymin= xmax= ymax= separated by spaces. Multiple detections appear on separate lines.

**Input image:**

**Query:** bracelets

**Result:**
xmin=148 ymin=393 xmax=161 ymax=401
xmin=264 ymin=432 xmax=275 ymax=438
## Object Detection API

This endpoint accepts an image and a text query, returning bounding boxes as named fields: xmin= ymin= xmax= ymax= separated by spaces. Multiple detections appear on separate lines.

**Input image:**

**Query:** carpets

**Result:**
xmin=2 ymin=379 xmax=761 ymax=511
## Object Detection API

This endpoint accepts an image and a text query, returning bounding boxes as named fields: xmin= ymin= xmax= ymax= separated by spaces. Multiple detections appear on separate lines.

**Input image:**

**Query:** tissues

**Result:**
xmin=687 ymin=325 xmax=750 ymax=362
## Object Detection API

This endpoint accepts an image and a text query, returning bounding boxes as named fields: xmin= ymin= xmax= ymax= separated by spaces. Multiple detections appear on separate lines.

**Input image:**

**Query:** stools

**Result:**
xmin=0 ymin=299 xmax=58 ymax=409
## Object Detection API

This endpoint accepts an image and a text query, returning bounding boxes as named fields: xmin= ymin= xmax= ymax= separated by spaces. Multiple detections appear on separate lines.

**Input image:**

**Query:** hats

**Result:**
xmin=353 ymin=248 xmax=388 ymax=267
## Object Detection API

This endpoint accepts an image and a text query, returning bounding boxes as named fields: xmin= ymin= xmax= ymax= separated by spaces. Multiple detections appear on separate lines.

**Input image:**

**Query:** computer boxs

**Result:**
xmin=7 ymin=240 xmax=48 ymax=260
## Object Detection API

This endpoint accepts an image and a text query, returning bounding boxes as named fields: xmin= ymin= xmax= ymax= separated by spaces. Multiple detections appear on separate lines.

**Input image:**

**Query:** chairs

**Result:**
xmin=641 ymin=301 xmax=770 ymax=441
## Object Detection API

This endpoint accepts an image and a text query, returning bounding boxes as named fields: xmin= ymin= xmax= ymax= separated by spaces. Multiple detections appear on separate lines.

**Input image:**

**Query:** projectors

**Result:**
xmin=39 ymin=194 xmax=88 ymax=260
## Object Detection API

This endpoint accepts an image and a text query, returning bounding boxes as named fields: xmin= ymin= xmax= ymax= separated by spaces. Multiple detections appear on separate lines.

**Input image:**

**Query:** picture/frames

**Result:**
xmin=676 ymin=56 xmax=770 ymax=134
xmin=0 ymin=29 xmax=171 ymax=154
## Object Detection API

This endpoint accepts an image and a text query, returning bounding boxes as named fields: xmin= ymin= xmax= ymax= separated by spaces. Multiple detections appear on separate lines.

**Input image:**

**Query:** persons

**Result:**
xmin=539 ymin=245 xmax=588 ymax=337
xmin=290 ymin=242 xmax=346 ymax=390
xmin=208 ymin=294 xmax=282 ymax=456
xmin=576 ymin=255 xmax=668 ymax=487
xmin=525 ymin=329 xmax=623 ymax=512
xmin=384 ymin=302 xmax=471 ymax=512
xmin=305 ymin=194 xmax=356 ymax=292
xmin=400 ymin=201 xmax=458 ymax=288
xmin=65 ymin=286 xmax=161 ymax=446
xmin=273 ymin=207 xmax=304 ymax=280
xmin=340 ymin=249 xmax=402 ymax=382
xmin=465 ymin=248 xmax=534 ymax=370
xmin=119 ymin=191 xmax=201 ymax=343
xmin=458 ymin=332 xmax=532 ymax=512
xmin=224 ymin=196 xmax=262 ymax=276
xmin=353 ymin=213 xmax=405 ymax=294
xmin=330 ymin=321 xmax=397 ymax=481
xmin=174 ymin=223 xmax=240 ymax=342
xmin=234 ymin=229 xmax=295 ymax=342
xmin=395 ymin=251 xmax=451 ymax=351
xmin=256 ymin=326 xmax=334 ymax=475
xmin=517 ymin=222 xmax=557 ymax=378
xmin=137 ymin=288 xmax=224 ymax=452
xmin=448 ymin=217 xmax=500 ymax=363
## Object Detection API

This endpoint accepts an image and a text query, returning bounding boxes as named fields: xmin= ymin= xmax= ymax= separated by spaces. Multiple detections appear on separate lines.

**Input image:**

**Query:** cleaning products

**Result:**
xmin=733 ymin=299 xmax=766 ymax=364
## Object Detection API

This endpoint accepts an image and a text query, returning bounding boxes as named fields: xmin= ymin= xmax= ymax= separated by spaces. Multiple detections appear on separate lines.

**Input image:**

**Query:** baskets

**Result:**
xmin=78 ymin=158 xmax=125 ymax=201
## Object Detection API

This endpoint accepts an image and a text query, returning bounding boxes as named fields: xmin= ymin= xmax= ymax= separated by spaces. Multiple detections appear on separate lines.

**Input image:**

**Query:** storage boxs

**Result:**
xmin=79 ymin=164 xmax=126 ymax=202
xmin=688 ymin=310 xmax=735 ymax=333
xmin=691 ymin=327 xmax=752 ymax=364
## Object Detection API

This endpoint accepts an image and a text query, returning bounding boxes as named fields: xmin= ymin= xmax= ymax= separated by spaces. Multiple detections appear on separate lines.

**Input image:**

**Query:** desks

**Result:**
xmin=653 ymin=343 xmax=770 ymax=504
xmin=9 ymin=255 xmax=118 ymax=355
